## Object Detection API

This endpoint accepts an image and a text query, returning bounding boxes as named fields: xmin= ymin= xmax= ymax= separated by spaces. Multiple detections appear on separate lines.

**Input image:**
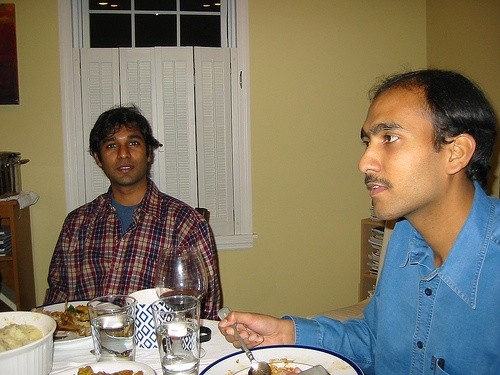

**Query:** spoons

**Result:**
xmin=217 ymin=307 xmax=271 ymax=375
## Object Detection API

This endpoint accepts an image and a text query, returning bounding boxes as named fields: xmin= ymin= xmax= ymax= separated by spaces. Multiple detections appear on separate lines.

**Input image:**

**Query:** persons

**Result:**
xmin=44 ymin=102 xmax=224 ymax=321
xmin=218 ymin=69 xmax=500 ymax=375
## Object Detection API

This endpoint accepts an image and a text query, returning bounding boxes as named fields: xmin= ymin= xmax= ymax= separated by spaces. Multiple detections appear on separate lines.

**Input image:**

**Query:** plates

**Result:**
xmin=43 ymin=301 xmax=92 ymax=344
xmin=199 ymin=345 xmax=364 ymax=375
xmin=53 ymin=360 xmax=156 ymax=375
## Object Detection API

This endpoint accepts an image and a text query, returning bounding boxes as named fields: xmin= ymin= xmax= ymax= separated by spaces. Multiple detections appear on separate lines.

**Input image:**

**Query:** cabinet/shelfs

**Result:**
xmin=359 ymin=218 xmax=386 ymax=304
xmin=0 ymin=200 xmax=36 ymax=311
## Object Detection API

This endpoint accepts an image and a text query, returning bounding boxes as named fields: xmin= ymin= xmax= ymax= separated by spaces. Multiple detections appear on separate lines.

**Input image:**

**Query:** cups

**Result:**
xmin=152 ymin=295 xmax=200 ymax=375
xmin=126 ymin=288 xmax=173 ymax=350
xmin=87 ymin=295 xmax=136 ymax=362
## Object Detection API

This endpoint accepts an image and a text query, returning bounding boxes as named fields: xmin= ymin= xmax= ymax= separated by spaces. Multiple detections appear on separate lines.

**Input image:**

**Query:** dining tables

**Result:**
xmin=49 ymin=318 xmax=242 ymax=375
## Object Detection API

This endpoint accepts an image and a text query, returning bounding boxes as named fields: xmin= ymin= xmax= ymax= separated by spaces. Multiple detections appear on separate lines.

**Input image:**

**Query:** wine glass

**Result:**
xmin=154 ymin=246 xmax=205 ymax=358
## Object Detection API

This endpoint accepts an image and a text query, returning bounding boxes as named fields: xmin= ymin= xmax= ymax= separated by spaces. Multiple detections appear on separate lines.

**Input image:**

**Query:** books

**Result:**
xmin=0 ymin=291 xmax=17 ymax=311
xmin=369 ymin=205 xmax=382 ymax=222
xmin=0 ymin=226 xmax=12 ymax=257
xmin=365 ymin=227 xmax=384 ymax=299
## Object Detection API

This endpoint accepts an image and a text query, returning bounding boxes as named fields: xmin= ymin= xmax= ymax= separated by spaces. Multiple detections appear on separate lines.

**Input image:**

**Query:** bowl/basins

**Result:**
xmin=0 ymin=311 xmax=57 ymax=375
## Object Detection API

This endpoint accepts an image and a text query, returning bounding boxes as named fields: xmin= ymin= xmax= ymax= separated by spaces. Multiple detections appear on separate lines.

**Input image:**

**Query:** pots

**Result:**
xmin=0 ymin=152 xmax=30 ymax=196
